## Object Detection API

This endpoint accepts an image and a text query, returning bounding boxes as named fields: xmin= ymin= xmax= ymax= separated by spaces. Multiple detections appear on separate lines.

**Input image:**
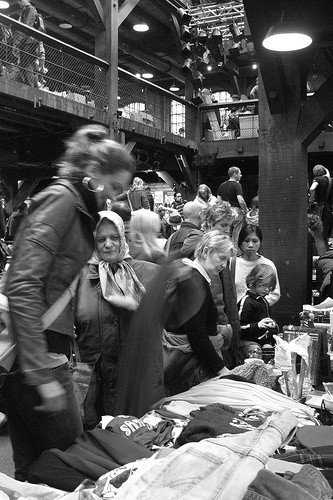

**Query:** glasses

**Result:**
xmin=259 ymin=283 xmax=272 ymax=290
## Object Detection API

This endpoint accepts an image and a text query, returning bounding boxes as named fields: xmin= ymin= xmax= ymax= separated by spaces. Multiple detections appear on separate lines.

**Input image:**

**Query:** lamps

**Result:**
xmin=58 ymin=19 xmax=72 ymax=29
xmin=261 ymin=10 xmax=313 ymax=52
xmin=177 ymin=7 xmax=244 ymax=89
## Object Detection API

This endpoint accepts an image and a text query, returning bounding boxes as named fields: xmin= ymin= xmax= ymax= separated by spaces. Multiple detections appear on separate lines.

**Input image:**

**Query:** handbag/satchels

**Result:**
xmin=0 ymin=292 xmax=19 ymax=373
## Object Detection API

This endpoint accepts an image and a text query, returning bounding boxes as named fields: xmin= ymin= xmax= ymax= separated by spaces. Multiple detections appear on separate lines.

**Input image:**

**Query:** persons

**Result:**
xmin=171 ymin=192 xmax=186 ymax=220
xmin=218 ymin=166 xmax=249 ymax=218
xmin=75 ymin=211 xmax=204 ymax=423
xmin=116 ymin=177 xmax=150 ymax=211
xmin=181 ymin=200 xmax=240 ymax=370
xmin=161 ymin=231 xmax=230 ymax=395
xmin=0 ymin=126 xmax=134 ymax=479
xmin=236 ymin=264 xmax=279 ymax=346
xmin=179 ymin=128 xmax=184 ymax=134
xmin=0 ymin=0 xmax=47 ymax=86
xmin=201 ymin=76 xmax=258 ymax=139
xmin=163 ymin=202 xmax=204 ymax=257
xmin=230 ymin=225 xmax=281 ymax=306
xmin=0 ymin=164 xmax=333 ymax=303
